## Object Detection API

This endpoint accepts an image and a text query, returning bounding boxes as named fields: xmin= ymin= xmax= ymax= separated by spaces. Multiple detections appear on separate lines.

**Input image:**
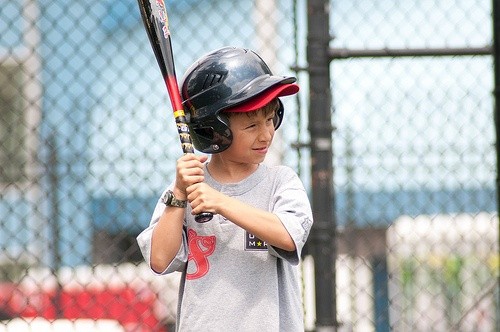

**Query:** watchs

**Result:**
xmin=163 ymin=189 xmax=187 ymax=208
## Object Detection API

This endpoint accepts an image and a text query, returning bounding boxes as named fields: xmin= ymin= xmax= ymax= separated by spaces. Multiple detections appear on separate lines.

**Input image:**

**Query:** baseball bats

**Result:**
xmin=138 ymin=0 xmax=214 ymax=223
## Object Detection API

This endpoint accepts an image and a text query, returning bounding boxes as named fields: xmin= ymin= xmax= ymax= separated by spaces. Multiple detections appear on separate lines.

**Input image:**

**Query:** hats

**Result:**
xmin=227 ymin=84 xmax=301 ymax=112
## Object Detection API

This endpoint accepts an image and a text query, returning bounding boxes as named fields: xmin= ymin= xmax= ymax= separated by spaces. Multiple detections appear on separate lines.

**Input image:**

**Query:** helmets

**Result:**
xmin=180 ymin=47 xmax=297 ymax=153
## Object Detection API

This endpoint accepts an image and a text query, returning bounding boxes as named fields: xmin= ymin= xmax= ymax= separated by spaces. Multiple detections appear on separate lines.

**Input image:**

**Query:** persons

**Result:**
xmin=136 ymin=45 xmax=314 ymax=332
